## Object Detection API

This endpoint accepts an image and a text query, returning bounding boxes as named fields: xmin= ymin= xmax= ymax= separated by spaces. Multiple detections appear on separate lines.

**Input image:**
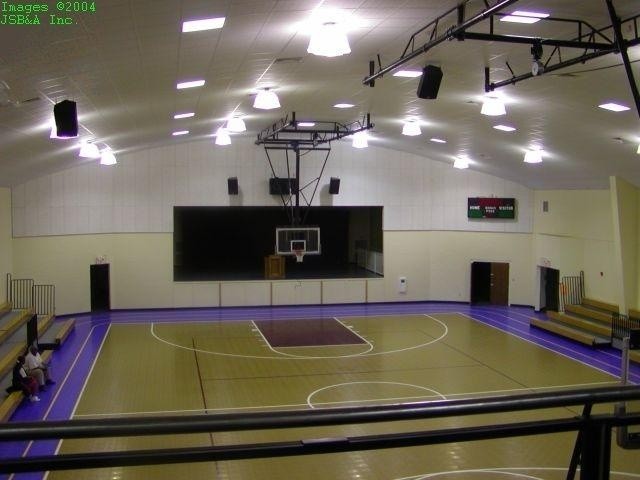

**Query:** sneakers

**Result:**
xmin=29 ymin=378 xmax=56 ymax=402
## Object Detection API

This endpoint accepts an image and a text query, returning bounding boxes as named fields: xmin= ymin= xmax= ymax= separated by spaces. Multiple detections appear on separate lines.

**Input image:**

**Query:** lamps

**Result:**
xmin=49 ymin=120 xmax=117 ymax=166
xmin=305 ymin=21 xmax=353 ymax=59
xmin=453 ymin=159 xmax=469 ymax=169
xmin=480 ymin=94 xmax=507 ymax=117
xmin=253 ymin=90 xmax=282 ymax=110
xmin=226 ymin=118 xmax=247 ymax=134
xmin=401 ymin=122 xmax=422 ymax=136
xmin=215 ymin=131 xmax=231 ymax=146
xmin=351 ymin=133 xmax=369 ymax=148
xmin=523 ymin=150 xmax=542 ymax=164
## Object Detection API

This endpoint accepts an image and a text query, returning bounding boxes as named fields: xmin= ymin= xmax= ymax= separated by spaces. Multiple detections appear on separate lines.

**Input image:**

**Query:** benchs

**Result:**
xmin=0 ymin=302 xmax=76 ymax=423
xmin=529 ymin=298 xmax=640 ymax=363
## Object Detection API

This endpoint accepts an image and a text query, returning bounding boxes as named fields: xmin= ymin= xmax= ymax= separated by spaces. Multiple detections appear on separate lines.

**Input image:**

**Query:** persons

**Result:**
xmin=11 ymin=345 xmax=56 ymax=403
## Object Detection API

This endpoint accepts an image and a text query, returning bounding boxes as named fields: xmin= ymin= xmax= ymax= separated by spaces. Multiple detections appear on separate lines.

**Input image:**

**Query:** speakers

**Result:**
xmin=330 ymin=177 xmax=340 ymax=193
xmin=54 ymin=100 xmax=77 ymax=136
xmin=228 ymin=177 xmax=238 ymax=194
xmin=269 ymin=178 xmax=299 ymax=195
